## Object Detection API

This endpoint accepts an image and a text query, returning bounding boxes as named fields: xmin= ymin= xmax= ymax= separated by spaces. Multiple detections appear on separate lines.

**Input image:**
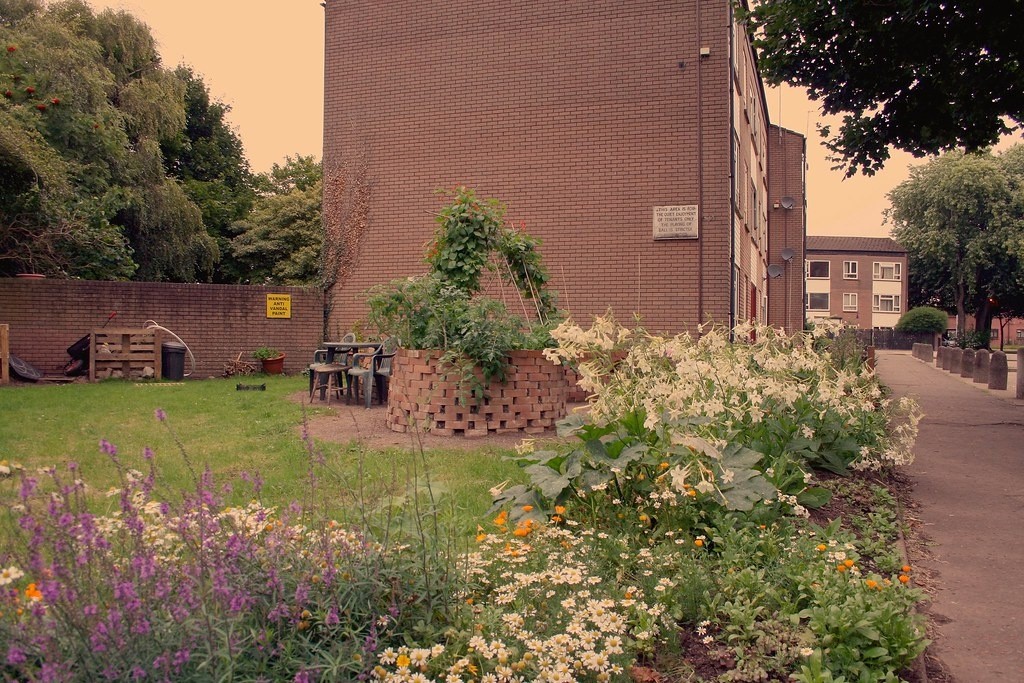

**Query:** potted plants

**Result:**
xmin=252 ymin=346 xmax=286 ymax=374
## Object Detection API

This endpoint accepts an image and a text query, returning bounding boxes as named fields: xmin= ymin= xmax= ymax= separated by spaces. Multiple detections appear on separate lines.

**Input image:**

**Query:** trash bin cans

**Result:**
xmin=160 ymin=341 xmax=187 ymax=384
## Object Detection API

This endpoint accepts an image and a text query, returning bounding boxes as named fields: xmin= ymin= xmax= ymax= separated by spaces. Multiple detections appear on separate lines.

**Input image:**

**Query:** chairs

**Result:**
xmin=308 ymin=333 xmax=356 ymax=401
xmin=347 ymin=337 xmax=397 ymax=408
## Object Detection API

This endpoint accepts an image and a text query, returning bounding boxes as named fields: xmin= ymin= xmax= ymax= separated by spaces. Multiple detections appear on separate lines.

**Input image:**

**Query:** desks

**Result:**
xmin=320 ymin=342 xmax=383 ymax=405
